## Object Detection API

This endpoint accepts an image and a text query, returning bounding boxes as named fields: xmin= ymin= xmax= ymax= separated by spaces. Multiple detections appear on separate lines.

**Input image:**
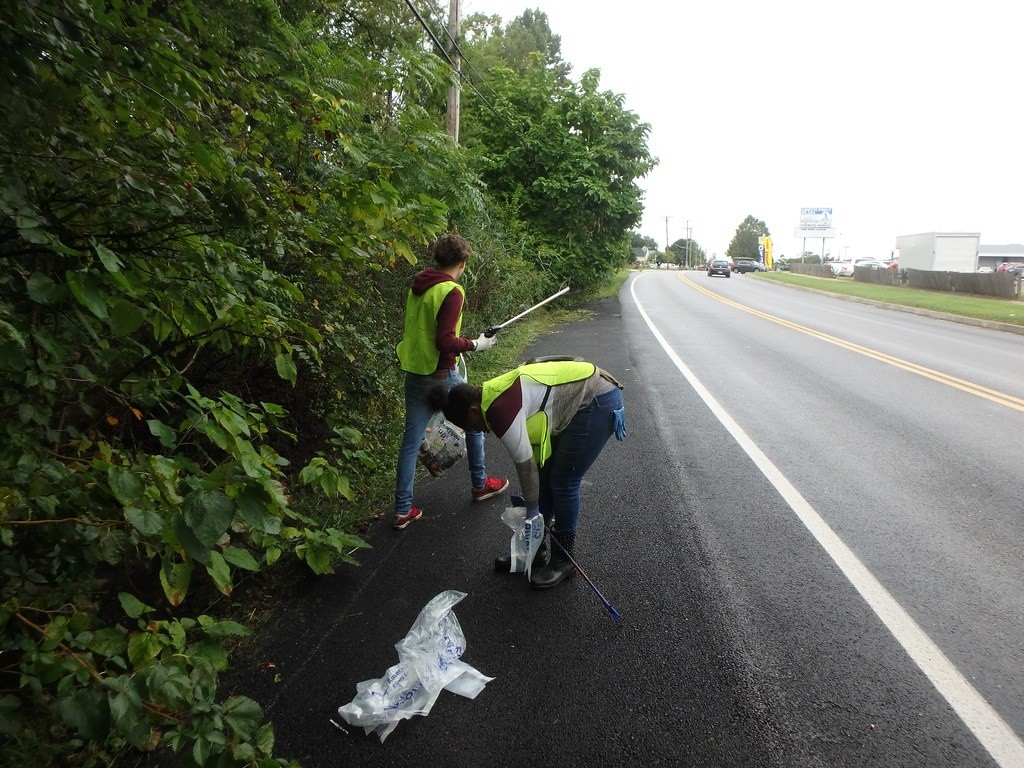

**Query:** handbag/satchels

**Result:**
xmin=417 ymin=408 xmax=468 ymax=478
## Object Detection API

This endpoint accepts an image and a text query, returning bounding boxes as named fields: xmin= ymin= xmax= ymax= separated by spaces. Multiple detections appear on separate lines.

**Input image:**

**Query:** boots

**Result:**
xmin=495 ymin=520 xmax=547 ymax=573
xmin=531 ymin=525 xmax=578 ymax=589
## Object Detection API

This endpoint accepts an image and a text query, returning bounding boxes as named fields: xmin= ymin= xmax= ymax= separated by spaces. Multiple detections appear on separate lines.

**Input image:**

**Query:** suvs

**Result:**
xmin=731 ymin=260 xmax=768 ymax=274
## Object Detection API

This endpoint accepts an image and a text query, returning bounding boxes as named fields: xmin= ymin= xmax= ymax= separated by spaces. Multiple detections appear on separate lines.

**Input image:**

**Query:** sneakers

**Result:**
xmin=471 ymin=477 xmax=509 ymax=503
xmin=393 ymin=507 xmax=423 ymax=529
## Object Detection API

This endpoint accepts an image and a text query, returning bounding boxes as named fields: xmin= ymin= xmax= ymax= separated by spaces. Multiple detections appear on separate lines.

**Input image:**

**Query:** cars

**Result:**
xmin=707 ymin=259 xmax=731 ymax=278
xmin=978 ymin=262 xmax=1024 ymax=281
xmin=824 ymin=256 xmax=898 ymax=277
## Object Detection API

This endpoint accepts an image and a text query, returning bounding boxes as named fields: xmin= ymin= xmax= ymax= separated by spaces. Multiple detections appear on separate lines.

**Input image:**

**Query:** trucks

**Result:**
xmin=889 ymin=232 xmax=980 ymax=278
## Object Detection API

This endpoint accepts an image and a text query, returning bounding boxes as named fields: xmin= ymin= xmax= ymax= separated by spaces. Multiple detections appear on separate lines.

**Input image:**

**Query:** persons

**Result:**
xmin=392 ymin=234 xmax=508 ymax=528
xmin=442 ymin=355 xmax=625 ymax=589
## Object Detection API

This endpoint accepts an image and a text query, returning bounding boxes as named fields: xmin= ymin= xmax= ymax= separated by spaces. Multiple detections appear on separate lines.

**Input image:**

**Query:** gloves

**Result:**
xmin=613 ymin=408 xmax=627 ymax=442
xmin=524 ymin=507 xmax=540 ymax=550
xmin=474 ymin=332 xmax=496 ymax=352
